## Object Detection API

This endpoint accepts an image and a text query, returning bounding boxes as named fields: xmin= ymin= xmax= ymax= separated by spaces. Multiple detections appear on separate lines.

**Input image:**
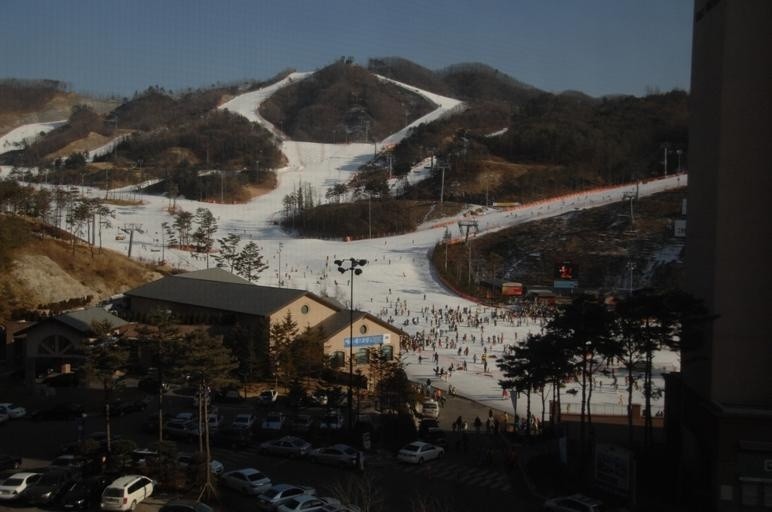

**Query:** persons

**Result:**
xmin=371 ymin=289 xmax=635 ymax=432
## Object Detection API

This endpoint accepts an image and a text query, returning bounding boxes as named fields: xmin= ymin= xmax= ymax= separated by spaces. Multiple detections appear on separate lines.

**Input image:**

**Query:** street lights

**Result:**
xmin=675 ymin=149 xmax=683 ymax=187
xmin=662 ymin=142 xmax=671 ymax=178
xmin=334 ymin=258 xmax=369 ymax=435
xmin=625 ymin=260 xmax=635 ymax=294
